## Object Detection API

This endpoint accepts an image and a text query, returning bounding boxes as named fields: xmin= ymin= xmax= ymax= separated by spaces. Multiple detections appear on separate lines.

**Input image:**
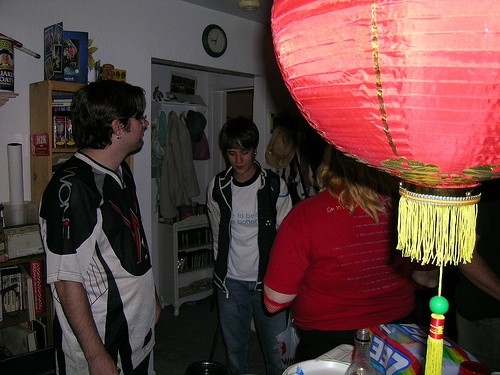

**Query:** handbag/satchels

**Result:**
xmin=190 ymin=132 xmax=210 ymax=160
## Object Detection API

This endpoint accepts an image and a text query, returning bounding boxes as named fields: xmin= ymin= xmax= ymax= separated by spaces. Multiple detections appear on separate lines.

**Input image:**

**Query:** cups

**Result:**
xmin=112 ymin=68 xmax=126 ymax=81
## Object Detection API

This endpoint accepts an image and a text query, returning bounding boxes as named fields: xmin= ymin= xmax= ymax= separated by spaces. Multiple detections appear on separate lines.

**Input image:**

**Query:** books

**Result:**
xmin=178 ymin=227 xmax=214 ymax=270
xmin=1 ymin=260 xmax=56 ymax=359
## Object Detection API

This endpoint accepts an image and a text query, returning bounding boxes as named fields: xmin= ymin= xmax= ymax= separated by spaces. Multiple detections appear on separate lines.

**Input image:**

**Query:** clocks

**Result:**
xmin=202 ymin=24 xmax=228 ymax=58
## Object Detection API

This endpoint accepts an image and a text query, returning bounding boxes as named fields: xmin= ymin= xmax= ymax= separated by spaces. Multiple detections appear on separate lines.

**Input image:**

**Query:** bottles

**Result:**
xmin=87 ymin=60 xmax=114 ymax=84
xmin=346 ymin=328 xmax=377 ymax=375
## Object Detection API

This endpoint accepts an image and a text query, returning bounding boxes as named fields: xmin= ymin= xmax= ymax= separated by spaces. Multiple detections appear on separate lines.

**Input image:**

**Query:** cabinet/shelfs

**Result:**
xmin=0 ymin=253 xmax=55 ymax=375
xmin=158 ymin=214 xmax=214 ymax=315
xmin=30 ymin=80 xmax=87 ymax=204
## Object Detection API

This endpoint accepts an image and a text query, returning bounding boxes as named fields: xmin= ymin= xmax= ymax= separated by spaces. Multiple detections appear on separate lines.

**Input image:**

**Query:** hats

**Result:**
xmin=187 ymin=110 xmax=207 ymax=137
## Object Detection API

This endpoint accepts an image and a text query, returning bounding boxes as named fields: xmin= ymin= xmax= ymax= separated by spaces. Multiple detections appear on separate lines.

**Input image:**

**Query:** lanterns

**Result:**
xmin=270 ymin=0 xmax=500 ymax=266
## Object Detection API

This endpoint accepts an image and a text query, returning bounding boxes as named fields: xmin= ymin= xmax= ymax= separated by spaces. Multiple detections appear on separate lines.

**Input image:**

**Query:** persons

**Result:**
xmin=38 ymin=79 xmax=161 ymax=374
xmin=206 ymin=117 xmax=295 ymax=375
xmin=262 ymin=144 xmax=500 ymax=363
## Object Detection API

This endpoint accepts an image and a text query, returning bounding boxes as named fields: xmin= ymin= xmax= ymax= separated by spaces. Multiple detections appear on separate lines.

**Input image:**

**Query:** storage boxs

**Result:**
xmin=372 ymin=323 xmax=479 ymax=375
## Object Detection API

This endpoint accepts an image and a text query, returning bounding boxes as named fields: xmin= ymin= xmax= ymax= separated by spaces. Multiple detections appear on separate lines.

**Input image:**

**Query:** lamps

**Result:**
xmin=239 ymin=0 xmax=261 ymax=11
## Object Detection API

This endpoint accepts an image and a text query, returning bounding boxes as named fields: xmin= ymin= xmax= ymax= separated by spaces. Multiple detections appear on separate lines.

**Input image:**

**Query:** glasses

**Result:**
xmin=123 ymin=115 xmax=147 ymax=125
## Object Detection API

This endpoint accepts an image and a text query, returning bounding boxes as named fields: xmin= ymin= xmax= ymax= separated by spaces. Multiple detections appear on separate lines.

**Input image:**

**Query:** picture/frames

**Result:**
xmin=169 ymin=71 xmax=198 ymax=95
xmin=2 ymin=223 xmax=46 ymax=260
xmin=63 ymin=31 xmax=88 ymax=83
